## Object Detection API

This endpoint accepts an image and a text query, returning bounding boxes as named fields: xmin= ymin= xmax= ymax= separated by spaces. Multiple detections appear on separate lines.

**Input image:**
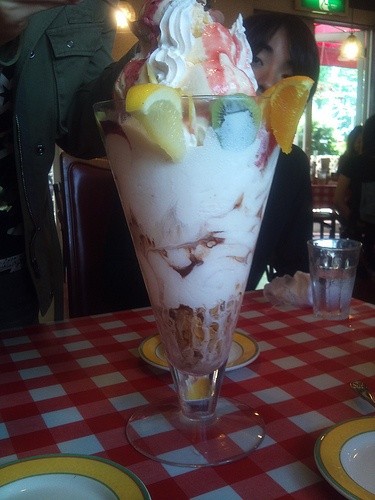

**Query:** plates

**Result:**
xmin=137 ymin=330 xmax=261 ymax=372
xmin=313 ymin=415 xmax=375 ymax=500
xmin=0 ymin=452 xmax=152 ymax=500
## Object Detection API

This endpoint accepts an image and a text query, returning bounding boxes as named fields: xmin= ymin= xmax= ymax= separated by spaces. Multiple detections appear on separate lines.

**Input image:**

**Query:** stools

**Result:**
xmin=312 ymin=211 xmax=335 ymax=240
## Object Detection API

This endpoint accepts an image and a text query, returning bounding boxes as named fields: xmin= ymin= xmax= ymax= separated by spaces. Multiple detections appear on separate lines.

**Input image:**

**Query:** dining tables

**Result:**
xmin=0 ymin=288 xmax=375 ymax=500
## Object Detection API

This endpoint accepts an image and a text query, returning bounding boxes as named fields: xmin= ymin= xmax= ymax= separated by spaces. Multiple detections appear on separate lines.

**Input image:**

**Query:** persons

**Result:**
xmin=1 ymin=0 xmax=121 ymax=326
xmin=89 ymin=0 xmax=316 ymax=293
xmin=335 ymin=116 xmax=375 ymax=292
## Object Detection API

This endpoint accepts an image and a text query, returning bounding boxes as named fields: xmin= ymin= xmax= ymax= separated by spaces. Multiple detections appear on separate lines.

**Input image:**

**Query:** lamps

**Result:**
xmin=341 ymin=0 xmax=360 ymax=60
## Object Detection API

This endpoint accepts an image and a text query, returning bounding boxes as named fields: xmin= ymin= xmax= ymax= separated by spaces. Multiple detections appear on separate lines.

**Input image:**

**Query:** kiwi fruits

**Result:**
xmin=211 ymin=92 xmax=261 ymax=148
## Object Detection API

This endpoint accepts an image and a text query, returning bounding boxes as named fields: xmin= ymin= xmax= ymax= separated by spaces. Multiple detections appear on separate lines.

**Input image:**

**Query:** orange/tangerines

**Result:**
xmin=124 ymin=85 xmax=187 ymax=159
xmin=259 ymin=76 xmax=313 ymax=152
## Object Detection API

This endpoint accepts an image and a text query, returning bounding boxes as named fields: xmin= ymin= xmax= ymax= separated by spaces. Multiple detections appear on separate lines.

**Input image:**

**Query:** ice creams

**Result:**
xmin=100 ymin=1 xmax=285 ymax=376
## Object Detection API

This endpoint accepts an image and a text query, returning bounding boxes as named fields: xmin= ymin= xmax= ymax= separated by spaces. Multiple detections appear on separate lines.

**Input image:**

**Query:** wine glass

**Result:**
xmin=90 ymin=92 xmax=281 ymax=468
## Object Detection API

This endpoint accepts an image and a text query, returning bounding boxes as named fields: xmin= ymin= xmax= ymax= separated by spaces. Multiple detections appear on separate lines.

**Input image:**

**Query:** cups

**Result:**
xmin=307 ymin=238 xmax=362 ymax=321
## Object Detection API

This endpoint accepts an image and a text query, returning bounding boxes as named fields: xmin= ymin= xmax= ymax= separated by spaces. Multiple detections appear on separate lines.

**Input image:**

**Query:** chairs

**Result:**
xmin=53 ymin=147 xmax=151 ymax=323
